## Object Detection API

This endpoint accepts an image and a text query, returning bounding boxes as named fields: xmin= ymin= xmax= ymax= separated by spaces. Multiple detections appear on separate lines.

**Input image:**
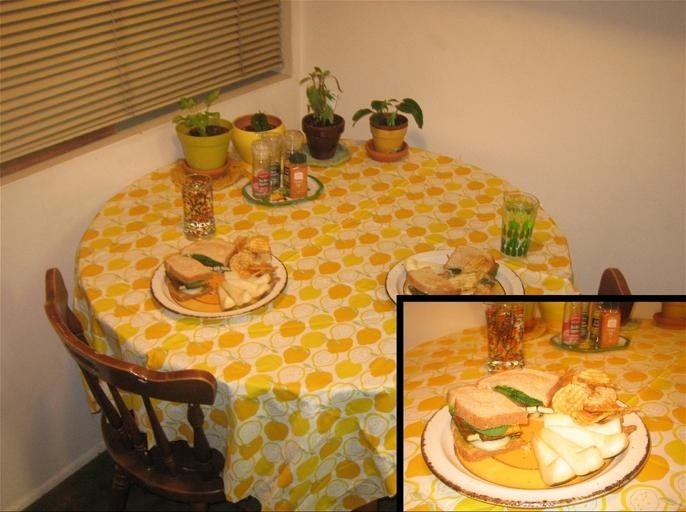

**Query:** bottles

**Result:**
xmin=561 ymin=302 xmax=622 ymax=351
xmin=252 ymin=131 xmax=308 ymax=200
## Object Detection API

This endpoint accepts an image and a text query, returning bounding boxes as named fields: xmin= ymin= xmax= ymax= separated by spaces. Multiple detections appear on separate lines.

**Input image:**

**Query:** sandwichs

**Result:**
xmin=165 ymin=237 xmax=234 ymax=301
xmin=401 ymin=243 xmax=500 ymax=296
xmin=445 ymin=368 xmax=560 ymax=462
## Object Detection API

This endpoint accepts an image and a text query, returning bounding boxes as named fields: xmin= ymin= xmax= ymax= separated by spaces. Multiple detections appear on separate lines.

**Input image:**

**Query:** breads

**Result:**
xmin=554 ymin=367 xmax=627 ymax=427
xmin=230 ymin=234 xmax=272 ymax=274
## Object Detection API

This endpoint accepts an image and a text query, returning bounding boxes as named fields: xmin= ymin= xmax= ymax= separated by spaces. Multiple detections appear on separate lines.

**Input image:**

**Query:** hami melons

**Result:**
xmin=217 ymin=270 xmax=271 ymax=310
xmin=533 ymin=410 xmax=629 ymax=487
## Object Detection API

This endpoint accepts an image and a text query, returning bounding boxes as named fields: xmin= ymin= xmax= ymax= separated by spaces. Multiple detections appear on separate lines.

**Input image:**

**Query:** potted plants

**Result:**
xmin=352 ymin=99 xmax=423 ymax=152
xmin=231 ymin=111 xmax=285 ymax=164
xmin=299 ymin=66 xmax=345 ymax=161
xmin=171 ymin=90 xmax=232 ymax=171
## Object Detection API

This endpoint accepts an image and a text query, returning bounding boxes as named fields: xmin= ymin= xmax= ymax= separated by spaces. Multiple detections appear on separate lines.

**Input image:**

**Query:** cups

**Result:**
xmin=180 ymin=174 xmax=216 ymax=239
xmin=483 ymin=302 xmax=526 ymax=374
xmin=499 ymin=190 xmax=540 ymax=258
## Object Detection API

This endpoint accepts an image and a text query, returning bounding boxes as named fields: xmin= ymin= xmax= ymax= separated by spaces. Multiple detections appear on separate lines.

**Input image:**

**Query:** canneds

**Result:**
xmin=599 ymin=302 xmax=621 ymax=348
xmin=288 ymin=153 xmax=308 ymax=199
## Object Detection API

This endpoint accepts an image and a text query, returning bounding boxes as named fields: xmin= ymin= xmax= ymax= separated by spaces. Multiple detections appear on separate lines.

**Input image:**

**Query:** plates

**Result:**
xmin=150 ymin=252 xmax=287 ymax=318
xmin=241 ymin=174 xmax=325 ymax=207
xmin=420 ymin=397 xmax=651 ymax=509
xmin=549 ymin=331 xmax=629 ymax=353
xmin=384 ymin=250 xmax=525 ymax=304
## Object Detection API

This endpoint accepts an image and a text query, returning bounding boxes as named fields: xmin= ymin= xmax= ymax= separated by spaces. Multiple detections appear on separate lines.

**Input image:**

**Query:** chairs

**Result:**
xmin=43 ymin=266 xmax=225 ymax=512
xmin=597 ymin=269 xmax=632 ymax=296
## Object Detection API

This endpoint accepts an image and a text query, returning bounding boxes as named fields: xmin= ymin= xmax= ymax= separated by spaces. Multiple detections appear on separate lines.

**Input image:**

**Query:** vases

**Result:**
xmin=488 ymin=302 xmax=686 ymax=333
